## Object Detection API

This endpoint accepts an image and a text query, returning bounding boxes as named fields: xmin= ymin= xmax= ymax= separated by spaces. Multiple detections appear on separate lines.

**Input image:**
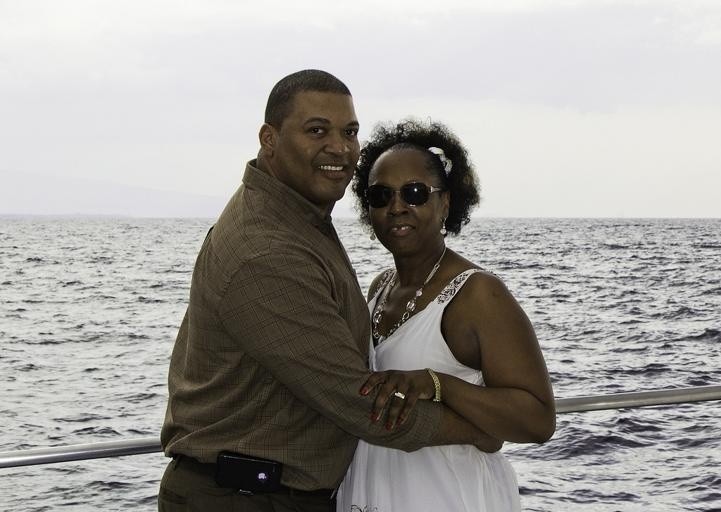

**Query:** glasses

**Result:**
xmin=365 ymin=181 xmax=441 ymax=209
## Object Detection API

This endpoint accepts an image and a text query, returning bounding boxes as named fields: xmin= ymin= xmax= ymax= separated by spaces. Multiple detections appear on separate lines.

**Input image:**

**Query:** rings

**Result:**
xmin=394 ymin=391 xmax=406 ymax=398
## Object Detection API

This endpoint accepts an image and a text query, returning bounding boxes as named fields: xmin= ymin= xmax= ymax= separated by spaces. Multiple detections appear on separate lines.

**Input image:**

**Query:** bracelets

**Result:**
xmin=426 ymin=369 xmax=444 ymax=404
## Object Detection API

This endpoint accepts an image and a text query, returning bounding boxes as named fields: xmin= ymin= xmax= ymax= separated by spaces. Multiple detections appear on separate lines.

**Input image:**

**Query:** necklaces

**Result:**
xmin=373 ymin=250 xmax=447 ymax=348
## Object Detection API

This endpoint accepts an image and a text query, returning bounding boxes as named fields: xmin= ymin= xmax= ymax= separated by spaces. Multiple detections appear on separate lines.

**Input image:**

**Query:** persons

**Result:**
xmin=155 ymin=68 xmax=504 ymax=511
xmin=336 ymin=119 xmax=556 ymax=509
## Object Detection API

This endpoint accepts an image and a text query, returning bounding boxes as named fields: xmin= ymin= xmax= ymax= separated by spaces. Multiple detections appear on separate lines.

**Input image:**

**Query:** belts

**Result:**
xmin=174 ymin=455 xmax=332 ymax=497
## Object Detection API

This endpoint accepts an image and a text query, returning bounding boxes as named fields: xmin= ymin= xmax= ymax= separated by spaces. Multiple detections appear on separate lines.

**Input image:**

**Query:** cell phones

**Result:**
xmin=215 ymin=450 xmax=282 ymax=494
